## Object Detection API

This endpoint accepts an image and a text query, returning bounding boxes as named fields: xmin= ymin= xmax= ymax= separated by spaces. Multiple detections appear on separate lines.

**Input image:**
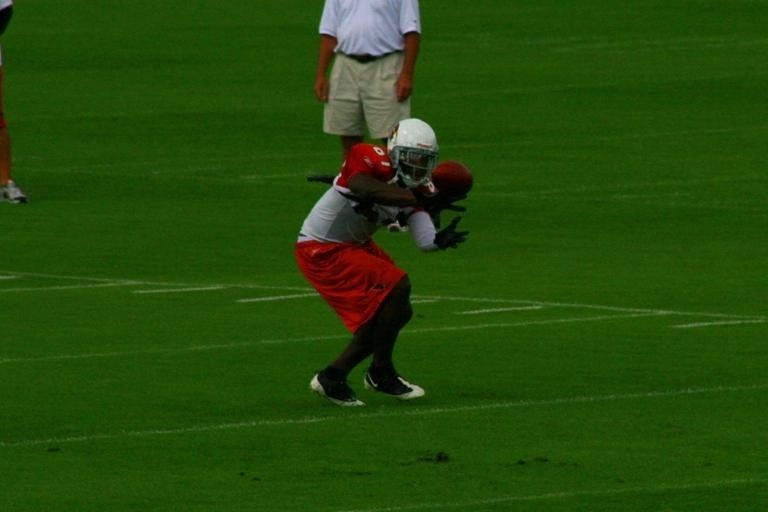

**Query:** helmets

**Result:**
xmin=387 ymin=118 xmax=439 ymax=188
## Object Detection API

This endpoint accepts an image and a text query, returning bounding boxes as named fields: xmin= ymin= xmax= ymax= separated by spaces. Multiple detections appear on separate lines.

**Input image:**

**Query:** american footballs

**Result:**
xmin=433 ymin=161 xmax=473 ymax=194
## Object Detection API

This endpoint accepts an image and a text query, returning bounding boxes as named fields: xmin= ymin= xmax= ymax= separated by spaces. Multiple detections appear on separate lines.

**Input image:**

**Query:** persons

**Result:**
xmin=315 ymin=1 xmax=424 ymax=233
xmin=0 ymin=1 xmax=29 ymax=207
xmin=292 ymin=116 xmax=475 ymax=406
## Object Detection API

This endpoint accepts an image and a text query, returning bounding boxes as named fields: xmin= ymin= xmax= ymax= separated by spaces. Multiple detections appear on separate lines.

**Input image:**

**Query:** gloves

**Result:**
xmin=424 ymin=186 xmax=467 ymax=228
xmin=435 ymin=217 xmax=470 ymax=249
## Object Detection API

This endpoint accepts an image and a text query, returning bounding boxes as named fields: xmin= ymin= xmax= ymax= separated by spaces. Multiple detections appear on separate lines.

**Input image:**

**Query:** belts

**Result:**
xmin=338 ymin=50 xmax=401 ymax=62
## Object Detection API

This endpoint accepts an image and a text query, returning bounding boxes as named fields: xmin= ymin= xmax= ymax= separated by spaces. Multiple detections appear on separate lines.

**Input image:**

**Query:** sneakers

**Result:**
xmin=363 ymin=370 xmax=424 ymax=399
xmin=0 ymin=180 xmax=27 ymax=204
xmin=310 ymin=374 xmax=367 ymax=407
